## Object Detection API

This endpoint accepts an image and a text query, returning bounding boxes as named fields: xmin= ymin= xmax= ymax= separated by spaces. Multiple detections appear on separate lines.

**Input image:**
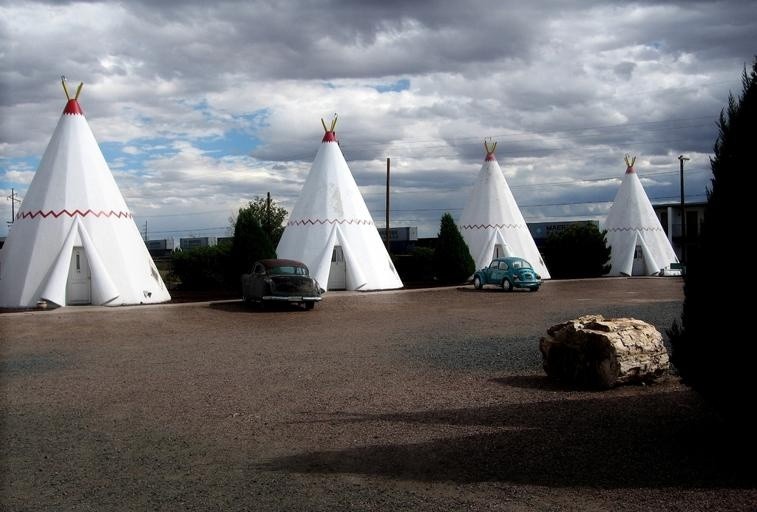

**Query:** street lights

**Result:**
xmin=676 ymin=155 xmax=690 ymax=263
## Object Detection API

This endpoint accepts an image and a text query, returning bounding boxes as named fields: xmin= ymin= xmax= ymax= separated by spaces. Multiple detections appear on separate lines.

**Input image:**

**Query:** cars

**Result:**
xmin=240 ymin=259 xmax=326 ymax=311
xmin=473 ymin=257 xmax=542 ymax=291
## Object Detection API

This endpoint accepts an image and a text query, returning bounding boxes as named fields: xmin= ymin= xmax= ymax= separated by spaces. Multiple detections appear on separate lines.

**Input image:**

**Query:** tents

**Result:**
xmin=457 ymin=154 xmax=552 ymax=282
xmin=600 ymin=165 xmax=688 ymax=277
xmin=0 ymin=100 xmax=175 ymax=309
xmin=270 ymin=131 xmax=405 ymax=292
